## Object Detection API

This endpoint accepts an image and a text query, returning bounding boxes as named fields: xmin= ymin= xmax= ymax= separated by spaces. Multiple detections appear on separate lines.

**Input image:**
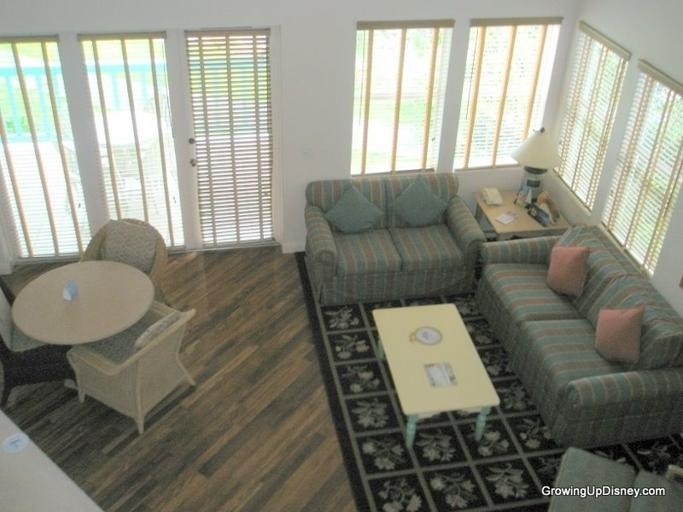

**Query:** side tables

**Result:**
xmin=474 ymin=189 xmax=570 ymax=240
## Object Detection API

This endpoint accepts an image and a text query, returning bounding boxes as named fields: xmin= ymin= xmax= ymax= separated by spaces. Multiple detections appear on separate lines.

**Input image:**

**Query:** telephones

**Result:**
xmin=480 ymin=187 xmax=503 ymax=205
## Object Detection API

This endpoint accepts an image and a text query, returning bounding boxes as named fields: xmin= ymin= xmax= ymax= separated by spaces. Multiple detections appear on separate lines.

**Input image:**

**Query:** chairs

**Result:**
xmin=66 ymin=300 xmax=196 ymax=434
xmin=77 ymin=217 xmax=170 ymax=309
xmin=0 ymin=276 xmax=73 ymax=409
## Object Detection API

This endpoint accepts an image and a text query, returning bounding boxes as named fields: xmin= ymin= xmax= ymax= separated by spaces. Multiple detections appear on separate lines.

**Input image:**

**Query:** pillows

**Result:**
xmin=388 ymin=174 xmax=449 ymax=228
xmin=547 ymin=245 xmax=590 ymax=298
xmin=323 ymin=184 xmax=384 ymax=234
xmin=595 ymin=305 xmax=644 ymax=367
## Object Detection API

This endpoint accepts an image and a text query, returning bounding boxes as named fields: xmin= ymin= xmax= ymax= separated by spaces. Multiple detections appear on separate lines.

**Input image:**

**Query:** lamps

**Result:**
xmin=509 ymin=126 xmax=563 ymax=208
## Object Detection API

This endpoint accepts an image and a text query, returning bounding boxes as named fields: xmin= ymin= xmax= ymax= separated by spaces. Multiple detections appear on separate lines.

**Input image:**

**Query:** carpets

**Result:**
xmin=294 ymin=235 xmax=682 ymax=512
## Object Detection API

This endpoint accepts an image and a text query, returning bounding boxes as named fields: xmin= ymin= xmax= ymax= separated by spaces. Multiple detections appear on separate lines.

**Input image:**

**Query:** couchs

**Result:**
xmin=305 ymin=172 xmax=486 ymax=307
xmin=547 ymin=447 xmax=683 ymax=509
xmin=475 ymin=222 xmax=683 ymax=450
